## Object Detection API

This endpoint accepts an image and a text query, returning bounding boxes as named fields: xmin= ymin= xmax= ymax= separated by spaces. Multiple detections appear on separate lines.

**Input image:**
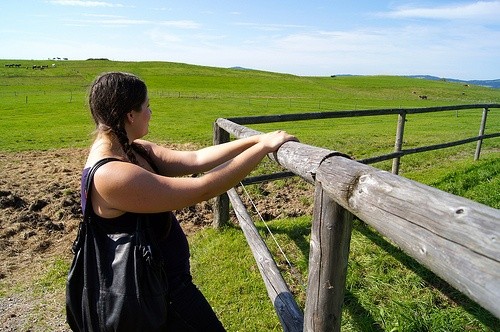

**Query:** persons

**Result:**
xmin=80 ymin=71 xmax=301 ymax=332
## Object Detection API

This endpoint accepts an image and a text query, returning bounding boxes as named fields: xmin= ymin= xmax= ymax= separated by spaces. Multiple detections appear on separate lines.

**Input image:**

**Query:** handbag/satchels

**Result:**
xmin=65 ymin=157 xmax=176 ymax=332
